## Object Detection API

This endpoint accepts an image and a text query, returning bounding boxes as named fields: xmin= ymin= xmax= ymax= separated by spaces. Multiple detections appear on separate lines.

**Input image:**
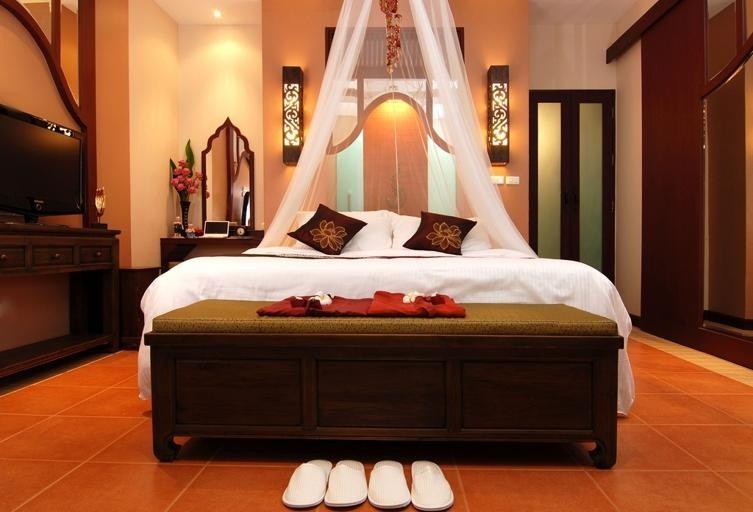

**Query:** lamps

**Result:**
xmin=486 ymin=65 xmax=509 ymax=167
xmin=282 ymin=65 xmax=304 ymax=166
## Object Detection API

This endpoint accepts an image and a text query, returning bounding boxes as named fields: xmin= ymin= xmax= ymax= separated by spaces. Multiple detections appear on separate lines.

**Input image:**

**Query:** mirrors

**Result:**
xmin=201 ymin=117 xmax=255 ymax=232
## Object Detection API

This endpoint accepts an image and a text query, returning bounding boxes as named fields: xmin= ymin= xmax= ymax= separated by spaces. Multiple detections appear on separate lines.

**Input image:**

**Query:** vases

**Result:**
xmin=180 ymin=201 xmax=191 ymax=237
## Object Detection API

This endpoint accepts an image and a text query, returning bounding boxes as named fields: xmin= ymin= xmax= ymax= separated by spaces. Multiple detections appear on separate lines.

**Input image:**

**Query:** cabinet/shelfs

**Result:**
xmin=0 ymin=234 xmax=119 ymax=380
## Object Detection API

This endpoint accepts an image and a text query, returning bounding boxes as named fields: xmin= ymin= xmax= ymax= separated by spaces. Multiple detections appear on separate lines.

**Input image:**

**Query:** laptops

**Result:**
xmin=198 ymin=220 xmax=230 ymax=238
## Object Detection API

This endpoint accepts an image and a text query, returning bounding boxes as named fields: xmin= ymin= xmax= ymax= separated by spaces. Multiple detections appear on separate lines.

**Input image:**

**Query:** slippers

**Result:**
xmin=324 ymin=460 xmax=368 ymax=508
xmin=411 ymin=461 xmax=454 ymax=512
xmin=369 ymin=460 xmax=412 ymax=510
xmin=281 ymin=460 xmax=333 ymax=508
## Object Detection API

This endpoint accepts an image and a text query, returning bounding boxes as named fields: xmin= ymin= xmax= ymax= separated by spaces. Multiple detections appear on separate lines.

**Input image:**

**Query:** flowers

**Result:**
xmin=169 ymin=139 xmax=202 ymax=201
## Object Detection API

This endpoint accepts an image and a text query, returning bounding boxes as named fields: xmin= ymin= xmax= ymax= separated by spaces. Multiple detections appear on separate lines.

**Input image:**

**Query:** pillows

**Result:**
xmin=287 ymin=203 xmax=491 ymax=255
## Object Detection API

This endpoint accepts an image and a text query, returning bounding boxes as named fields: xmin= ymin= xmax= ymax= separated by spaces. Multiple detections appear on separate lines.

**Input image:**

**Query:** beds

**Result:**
xmin=137 ymin=245 xmax=633 ymax=416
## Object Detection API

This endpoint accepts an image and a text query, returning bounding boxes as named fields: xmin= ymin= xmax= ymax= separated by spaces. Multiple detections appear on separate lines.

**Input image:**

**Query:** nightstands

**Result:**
xmin=160 ymin=230 xmax=265 ymax=274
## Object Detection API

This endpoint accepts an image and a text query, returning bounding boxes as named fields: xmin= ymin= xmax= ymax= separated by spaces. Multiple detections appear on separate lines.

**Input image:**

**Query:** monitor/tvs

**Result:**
xmin=1 ymin=104 xmax=87 ymax=228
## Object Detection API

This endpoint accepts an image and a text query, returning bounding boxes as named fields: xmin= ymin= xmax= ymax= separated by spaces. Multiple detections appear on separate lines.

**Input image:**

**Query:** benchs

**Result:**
xmin=144 ymin=300 xmax=624 ymax=469
